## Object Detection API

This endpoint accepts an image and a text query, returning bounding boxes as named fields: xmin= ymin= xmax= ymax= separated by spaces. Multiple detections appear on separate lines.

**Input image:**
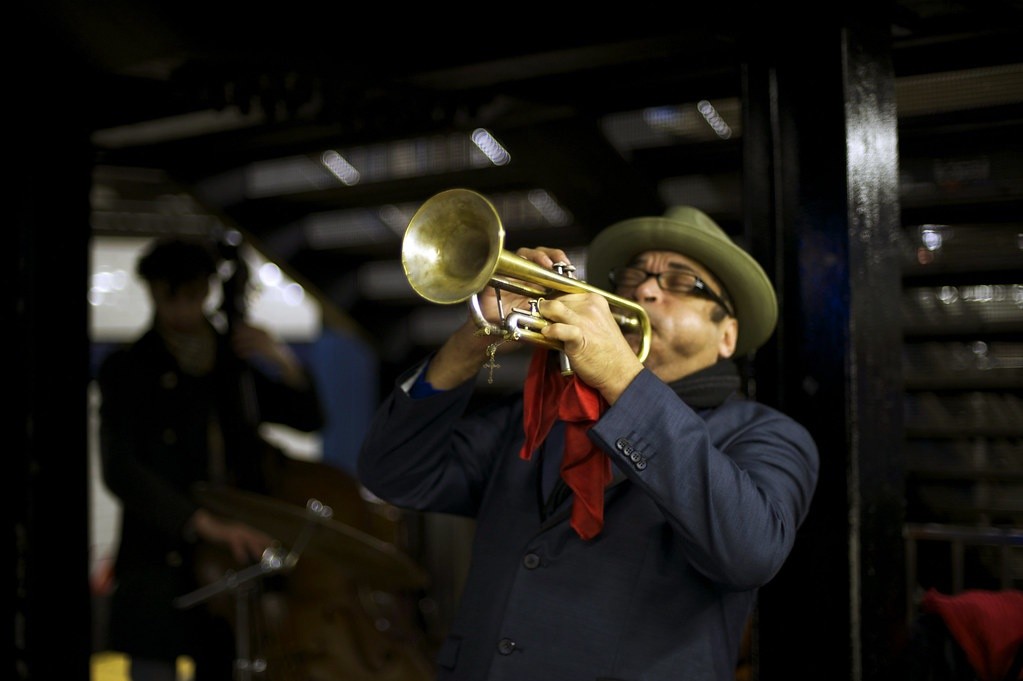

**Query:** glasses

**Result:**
xmin=608 ymin=266 xmax=731 ymax=317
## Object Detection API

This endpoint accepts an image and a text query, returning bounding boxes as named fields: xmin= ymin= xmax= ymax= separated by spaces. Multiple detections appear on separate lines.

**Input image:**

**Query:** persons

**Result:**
xmin=361 ymin=201 xmax=819 ymax=680
xmin=81 ymin=203 xmax=325 ymax=681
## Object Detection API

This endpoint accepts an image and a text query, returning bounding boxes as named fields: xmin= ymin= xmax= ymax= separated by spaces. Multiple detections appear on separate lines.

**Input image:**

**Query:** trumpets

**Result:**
xmin=401 ymin=188 xmax=649 ymax=382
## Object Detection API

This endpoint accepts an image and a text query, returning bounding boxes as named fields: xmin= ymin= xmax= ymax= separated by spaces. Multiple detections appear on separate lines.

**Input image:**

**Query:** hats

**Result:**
xmin=585 ymin=205 xmax=777 ymax=355
